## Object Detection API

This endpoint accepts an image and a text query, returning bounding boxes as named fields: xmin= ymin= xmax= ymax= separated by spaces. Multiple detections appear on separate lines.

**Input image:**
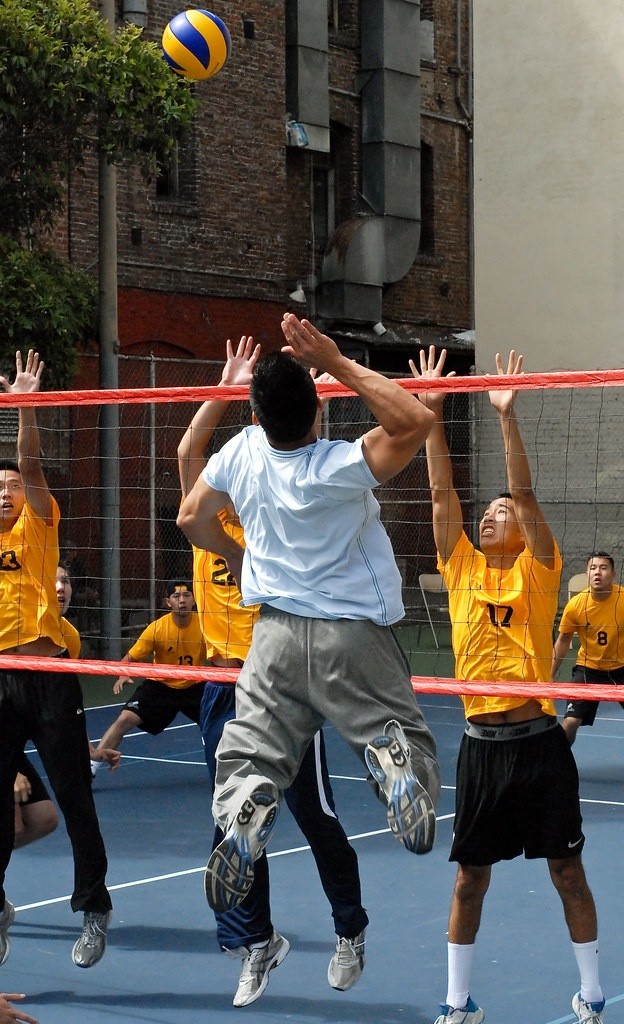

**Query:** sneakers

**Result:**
xmin=203 ymin=773 xmax=281 ymax=913
xmin=433 ymin=991 xmax=486 ymax=1024
xmin=327 ymin=931 xmax=367 ymax=992
xmin=222 ymin=929 xmax=292 ymax=1008
xmin=70 ymin=910 xmax=113 ymax=969
xmin=571 ymin=991 xmax=607 ymax=1024
xmin=363 ymin=718 xmax=438 ymax=855
xmin=0 ymin=899 xmax=16 ymax=967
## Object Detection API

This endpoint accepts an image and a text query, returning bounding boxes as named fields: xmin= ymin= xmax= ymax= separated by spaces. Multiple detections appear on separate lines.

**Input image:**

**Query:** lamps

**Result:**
xmin=282 ymin=277 xmax=307 ymax=302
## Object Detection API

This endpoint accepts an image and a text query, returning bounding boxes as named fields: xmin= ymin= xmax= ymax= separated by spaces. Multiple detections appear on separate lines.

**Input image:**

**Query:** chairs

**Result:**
xmin=565 ymin=573 xmax=588 ymax=649
xmin=415 ymin=573 xmax=452 ymax=649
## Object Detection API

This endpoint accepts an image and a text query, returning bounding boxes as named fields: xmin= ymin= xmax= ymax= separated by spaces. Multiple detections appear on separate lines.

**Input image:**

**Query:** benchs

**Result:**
xmin=64 ymin=605 xmax=143 ymax=640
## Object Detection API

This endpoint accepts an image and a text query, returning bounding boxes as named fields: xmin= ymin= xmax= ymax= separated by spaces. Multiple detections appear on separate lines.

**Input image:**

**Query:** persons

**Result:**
xmin=0 ymin=349 xmax=113 ymax=1024
xmin=178 ymin=335 xmax=369 ymax=1007
xmin=174 ymin=313 xmax=442 ymax=913
xmin=551 ymin=551 xmax=624 ymax=747
xmin=91 ymin=582 xmax=208 ymax=785
xmin=408 ymin=346 xmax=604 ymax=1024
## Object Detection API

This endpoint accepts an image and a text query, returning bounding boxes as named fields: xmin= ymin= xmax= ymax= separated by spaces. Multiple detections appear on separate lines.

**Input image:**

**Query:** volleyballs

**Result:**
xmin=162 ymin=9 xmax=231 ymax=80
